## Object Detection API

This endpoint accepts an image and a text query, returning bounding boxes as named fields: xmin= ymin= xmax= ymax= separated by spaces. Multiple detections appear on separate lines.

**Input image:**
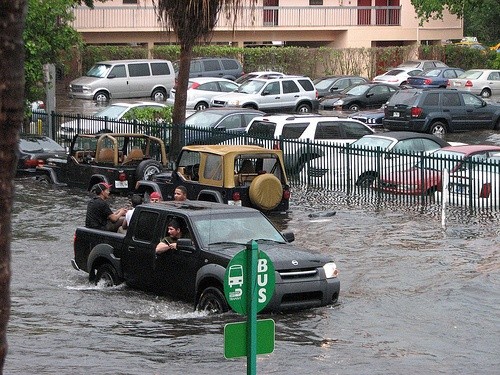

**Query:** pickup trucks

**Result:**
xmin=70 ymin=200 xmax=340 ymax=315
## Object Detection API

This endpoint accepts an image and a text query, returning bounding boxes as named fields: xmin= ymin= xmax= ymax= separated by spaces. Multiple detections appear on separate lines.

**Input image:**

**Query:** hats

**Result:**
xmin=150 ymin=192 xmax=161 ymax=199
xmin=95 ymin=182 xmax=112 ymax=195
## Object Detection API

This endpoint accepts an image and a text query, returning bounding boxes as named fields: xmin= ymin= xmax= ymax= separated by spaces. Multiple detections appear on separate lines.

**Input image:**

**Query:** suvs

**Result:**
xmin=215 ymin=113 xmax=378 ymax=174
xmin=34 ymin=131 xmax=170 ymax=197
xmin=382 ymin=88 xmax=500 ymax=140
xmin=134 ymin=144 xmax=290 ymax=214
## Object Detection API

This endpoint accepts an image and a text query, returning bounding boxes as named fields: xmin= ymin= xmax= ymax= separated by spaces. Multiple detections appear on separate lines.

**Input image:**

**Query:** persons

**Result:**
xmin=117 ymin=195 xmax=142 ymax=235
xmin=86 ymin=182 xmax=128 ymax=232
xmin=149 ymin=192 xmax=162 ymax=203
xmin=155 ymin=219 xmax=193 ymax=254
xmin=174 ymin=185 xmax=190 ymax=201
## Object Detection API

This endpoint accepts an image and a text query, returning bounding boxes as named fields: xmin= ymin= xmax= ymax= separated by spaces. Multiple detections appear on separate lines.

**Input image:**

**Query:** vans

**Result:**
xmin=68 ymin=58 xmax=176 ymax=103
xmin=175 ymin=56 xmax=245 ymax=81
xmin=208 ymin=71 xmax=320 ymax=114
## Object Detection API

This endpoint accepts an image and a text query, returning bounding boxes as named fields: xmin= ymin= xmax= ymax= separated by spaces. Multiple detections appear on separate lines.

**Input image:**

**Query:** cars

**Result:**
xmin=315 ymin=75 xmax=403 ymax=128
xmin=142 ymin=107 xmax=269 ymax=159
xmin=371 ymin=60 xmax=500 ymax=98
xmin=298 ymin=130 xmax=500 ymax=206
xmin=15 ymin=134 xmax=68 ymax=187
xmin=440 ymin=37 xmax=500 ymax=59
xmin=169 ymin=76 xmax=242 ymax=111
xmin=57 ymin=101 xmax=175 ymax=142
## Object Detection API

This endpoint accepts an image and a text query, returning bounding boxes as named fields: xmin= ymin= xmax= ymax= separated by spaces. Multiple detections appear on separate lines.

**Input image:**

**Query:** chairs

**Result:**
xmin=237 ymin=159 xmax=254 ymax=173
xmin=98 ymin=148 xmax=114 ymax=162
xmin=122 ymin=150 xmax=143 ymax=163
